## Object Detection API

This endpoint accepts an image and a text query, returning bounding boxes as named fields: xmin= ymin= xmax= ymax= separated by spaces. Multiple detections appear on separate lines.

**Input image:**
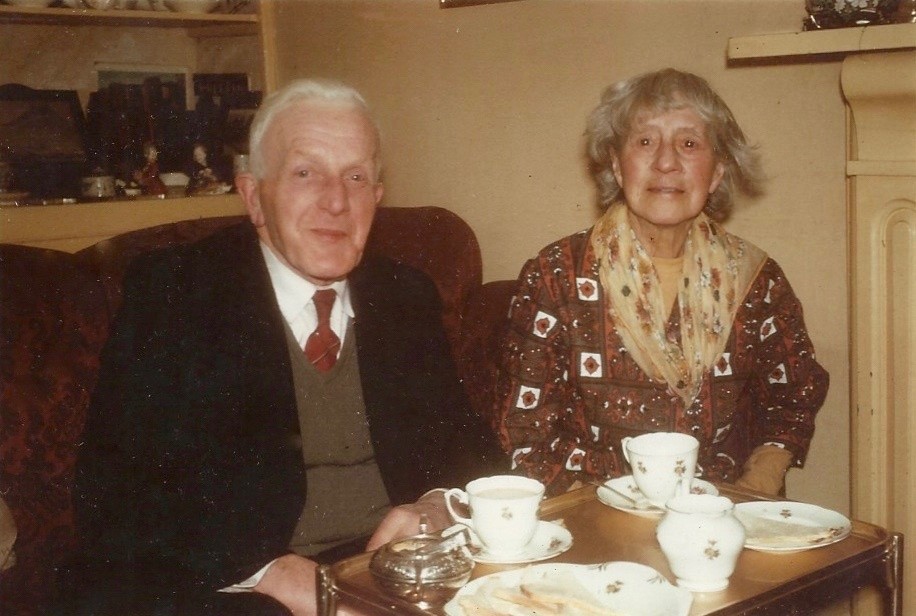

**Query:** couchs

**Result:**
xmin=0 ymin=205 xmax=530 ymax=615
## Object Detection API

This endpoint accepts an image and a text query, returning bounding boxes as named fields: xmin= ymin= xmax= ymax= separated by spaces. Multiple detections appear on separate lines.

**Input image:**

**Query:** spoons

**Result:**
xmin=578 ymin=475 xmax=668 ymax=512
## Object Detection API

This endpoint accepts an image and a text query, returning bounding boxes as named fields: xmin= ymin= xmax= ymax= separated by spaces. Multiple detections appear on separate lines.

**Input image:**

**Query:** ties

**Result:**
xmin=305 ymin=289 xmax=342 ymax=373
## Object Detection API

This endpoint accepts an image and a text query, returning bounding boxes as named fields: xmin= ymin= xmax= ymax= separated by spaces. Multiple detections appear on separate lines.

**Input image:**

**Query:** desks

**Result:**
xmin=313 ymin=473 xmax=905 ymax=616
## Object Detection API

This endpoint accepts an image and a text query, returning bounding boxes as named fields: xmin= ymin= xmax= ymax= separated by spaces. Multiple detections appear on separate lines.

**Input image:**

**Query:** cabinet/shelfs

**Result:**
xmin=0 ymin=0 xmax=292 ymax=266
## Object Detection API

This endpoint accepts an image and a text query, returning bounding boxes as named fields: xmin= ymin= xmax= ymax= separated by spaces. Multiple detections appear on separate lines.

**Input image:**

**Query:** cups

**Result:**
xmin=621 ymin=430 xmax=700 ymax=500
xmin=446 ymin=477 xmax=544 ymax=553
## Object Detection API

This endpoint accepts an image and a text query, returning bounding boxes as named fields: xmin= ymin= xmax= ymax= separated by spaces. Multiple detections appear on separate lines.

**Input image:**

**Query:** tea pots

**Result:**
xmin=367 ymin=513 xmax=475 ymax=611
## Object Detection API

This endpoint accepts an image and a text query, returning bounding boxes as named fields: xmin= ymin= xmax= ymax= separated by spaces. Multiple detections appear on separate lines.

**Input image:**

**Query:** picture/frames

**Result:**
xmin=1 ymin=58 xmax=269 ymax=200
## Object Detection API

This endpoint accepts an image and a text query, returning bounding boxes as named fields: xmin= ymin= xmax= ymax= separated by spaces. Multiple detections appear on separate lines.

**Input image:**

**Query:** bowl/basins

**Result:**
xmin=658 ymin=493 xmax=747 ymax=592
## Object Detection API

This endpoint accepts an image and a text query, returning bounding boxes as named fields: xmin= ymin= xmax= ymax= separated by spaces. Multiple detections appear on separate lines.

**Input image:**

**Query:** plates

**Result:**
xmin=732 ymin=500 xmax=853 ymax=554
xmin=598 ymin=474 xmax=719 ymax=519
xmin=445 ymin=560 xmax=695 ymax=615
xmin=444 ymin=520 xmax=577 ymax=565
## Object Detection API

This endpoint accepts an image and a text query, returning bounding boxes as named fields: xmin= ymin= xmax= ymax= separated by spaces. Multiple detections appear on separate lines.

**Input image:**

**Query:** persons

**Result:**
xmin=494 ymin=59 xmax=829 ymax=512
xmin=127 ymin=138 xmax=219 ymax=196
xmin=58 ymin=76 xmax=511 ymax=616
